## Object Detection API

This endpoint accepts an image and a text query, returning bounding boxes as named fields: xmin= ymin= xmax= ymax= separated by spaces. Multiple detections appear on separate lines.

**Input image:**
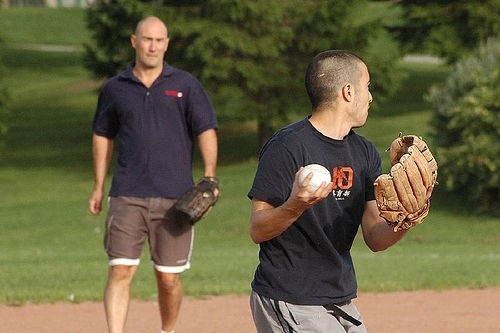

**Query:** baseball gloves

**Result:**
xmin=174 ymin=177 xmax=221 ymax=223
xmin=373 ymin=134 xmax=438 ymax=232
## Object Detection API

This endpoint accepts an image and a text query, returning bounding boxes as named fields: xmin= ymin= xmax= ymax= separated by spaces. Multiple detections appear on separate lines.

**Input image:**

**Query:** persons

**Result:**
xmin=86 ymin=15 xmax=219 ymax=333
xmin=247 ymin=50 xmax=417 ymax=333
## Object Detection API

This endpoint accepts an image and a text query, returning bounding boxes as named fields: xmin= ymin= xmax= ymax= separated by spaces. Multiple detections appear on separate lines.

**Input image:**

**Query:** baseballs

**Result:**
xmin=299 ymin=164 xmax=333 ymax=193
